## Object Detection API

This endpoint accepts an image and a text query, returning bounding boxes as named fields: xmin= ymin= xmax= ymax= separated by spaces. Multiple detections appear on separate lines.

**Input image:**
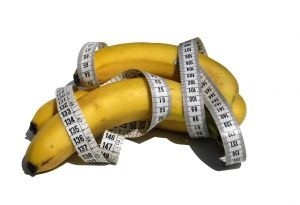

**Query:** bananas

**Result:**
xmin=25 ymin=43 xmax=246 ymax=177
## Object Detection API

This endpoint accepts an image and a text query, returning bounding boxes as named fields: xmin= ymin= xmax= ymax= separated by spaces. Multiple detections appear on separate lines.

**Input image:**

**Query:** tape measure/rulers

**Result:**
xmin=56 ymin=37 xmax=246 ymax=166
